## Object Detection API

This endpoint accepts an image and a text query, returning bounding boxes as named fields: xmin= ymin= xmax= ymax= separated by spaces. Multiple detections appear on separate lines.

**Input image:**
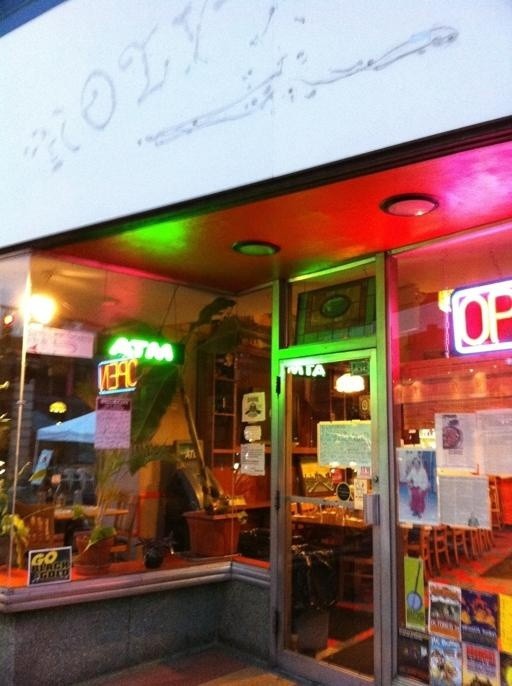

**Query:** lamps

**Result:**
xmin=379 ymin=193 xmax=440 ymax=217
xmin=232 ymin=240 xmax=281 ymax=257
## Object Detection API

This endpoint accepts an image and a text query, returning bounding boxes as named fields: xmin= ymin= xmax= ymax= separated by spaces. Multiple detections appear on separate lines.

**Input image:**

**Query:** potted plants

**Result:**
xmin=73 ymin=442 xmax=176 ymax=576
xmin=131 ymin=299 xmax=248 ymax=557
xmin=292 ymin=551 xmax=335 ymax=651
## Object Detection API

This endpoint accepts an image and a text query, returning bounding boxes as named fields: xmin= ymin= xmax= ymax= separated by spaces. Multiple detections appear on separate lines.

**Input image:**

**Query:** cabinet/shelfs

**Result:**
xmin=195 ymin=339 xmax=270 ymax=546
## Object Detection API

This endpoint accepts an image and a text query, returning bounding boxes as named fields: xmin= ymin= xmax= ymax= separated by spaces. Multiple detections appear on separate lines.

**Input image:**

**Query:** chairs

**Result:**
xmin=13 ymin=504 xmax=54 ymax=550
xmin=448 ymin=529 xmax=469 ymax=566
xmin=110 ymin=490 xmax=140 ymax=563
xmin=408 ymin=526 xmax=433 ymax=586
xmin=339 ymin=551 xmax=376 ymax=612
xmin=431 ymin=525 xmax=451 ymax=577
